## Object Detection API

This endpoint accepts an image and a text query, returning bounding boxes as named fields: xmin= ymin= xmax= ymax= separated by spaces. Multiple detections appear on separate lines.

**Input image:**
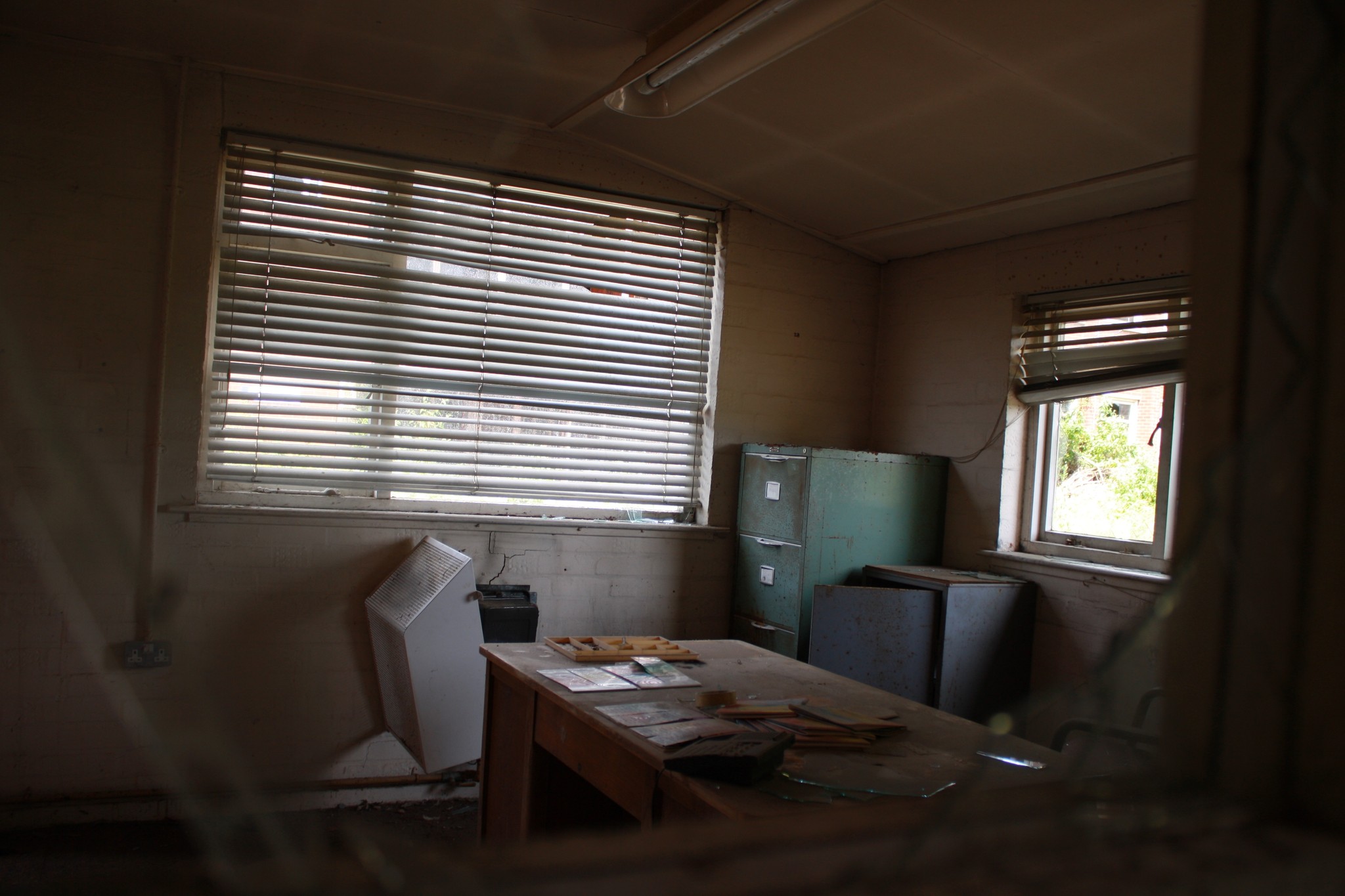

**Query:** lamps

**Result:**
xmin=602 ymin=0 xmax=884 ymax=120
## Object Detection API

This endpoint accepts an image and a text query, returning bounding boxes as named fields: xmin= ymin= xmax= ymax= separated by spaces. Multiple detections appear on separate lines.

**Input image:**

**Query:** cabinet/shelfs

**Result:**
xmin=727 ymin=438 xmax=953 ymax=665
xmin=804 ymin=562 xmax=1038 ymax=742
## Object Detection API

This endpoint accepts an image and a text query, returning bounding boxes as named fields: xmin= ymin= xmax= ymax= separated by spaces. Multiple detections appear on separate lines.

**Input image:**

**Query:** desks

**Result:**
xmin=476 ymin=637 xmax=1070 ymax=852
